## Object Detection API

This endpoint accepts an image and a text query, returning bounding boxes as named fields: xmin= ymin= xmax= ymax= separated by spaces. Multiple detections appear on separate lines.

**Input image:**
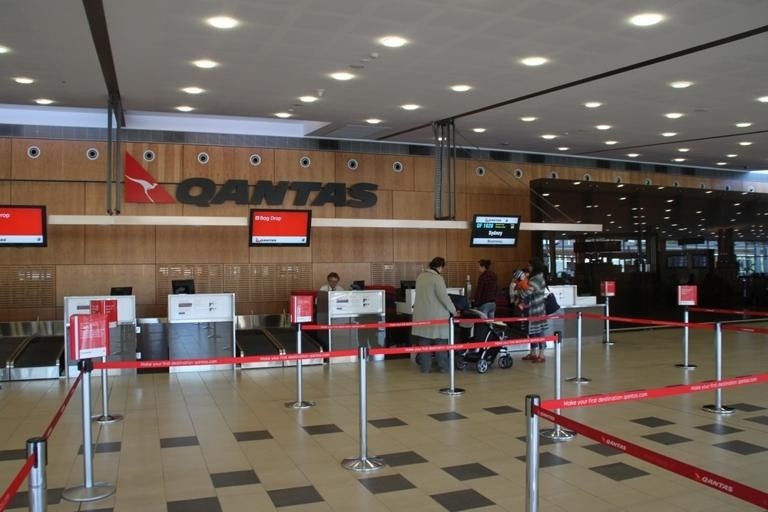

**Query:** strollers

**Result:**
xmin=455 ymin=309 xmax=514 ymax=374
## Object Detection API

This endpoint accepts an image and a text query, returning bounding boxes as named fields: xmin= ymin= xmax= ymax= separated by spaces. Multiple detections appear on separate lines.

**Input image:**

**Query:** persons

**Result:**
xmin=471 ymin=259 xmax=498 ymax=328
xmin=411 ymin=257 xmax=461 ymax=373
xmin=510 ymin=257 xmax=549 ymax=364
xmin=314 ymin=271 xmax=345 ymax=308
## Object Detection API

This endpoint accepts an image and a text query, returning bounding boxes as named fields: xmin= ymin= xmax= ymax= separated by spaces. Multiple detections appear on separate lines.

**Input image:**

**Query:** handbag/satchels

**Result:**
xmin=544 ymin=293 xmax=560 ymax=315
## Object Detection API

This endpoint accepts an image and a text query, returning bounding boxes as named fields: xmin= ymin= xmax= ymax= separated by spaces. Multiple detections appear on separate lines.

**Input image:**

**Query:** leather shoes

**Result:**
xmin=521 ymin=354 xmax=546 ymax=363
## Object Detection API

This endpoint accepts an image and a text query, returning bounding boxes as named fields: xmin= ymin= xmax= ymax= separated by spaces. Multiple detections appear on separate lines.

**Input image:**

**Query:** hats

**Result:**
xmin=513 ymin=269 xmax=525 ymax=279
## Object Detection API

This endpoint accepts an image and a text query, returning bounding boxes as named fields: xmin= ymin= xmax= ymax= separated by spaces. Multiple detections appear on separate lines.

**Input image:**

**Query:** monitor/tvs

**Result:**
xmin=470 ymin=214 xmax=521 ymax=248
xmin=353 ymin=280 xmax=364 ymax=290
xmin=111 ymin=287 xmax=132 ymax=296
xmin=249 ymin=209 xmax=312 ymax=247
xmin=0 ymin=205 xmax=47 ymax=247
xmin=172 ymin=279 xmax=195 ymax=294
xmin=400 ymin=280 xmax=416 ymax=302
